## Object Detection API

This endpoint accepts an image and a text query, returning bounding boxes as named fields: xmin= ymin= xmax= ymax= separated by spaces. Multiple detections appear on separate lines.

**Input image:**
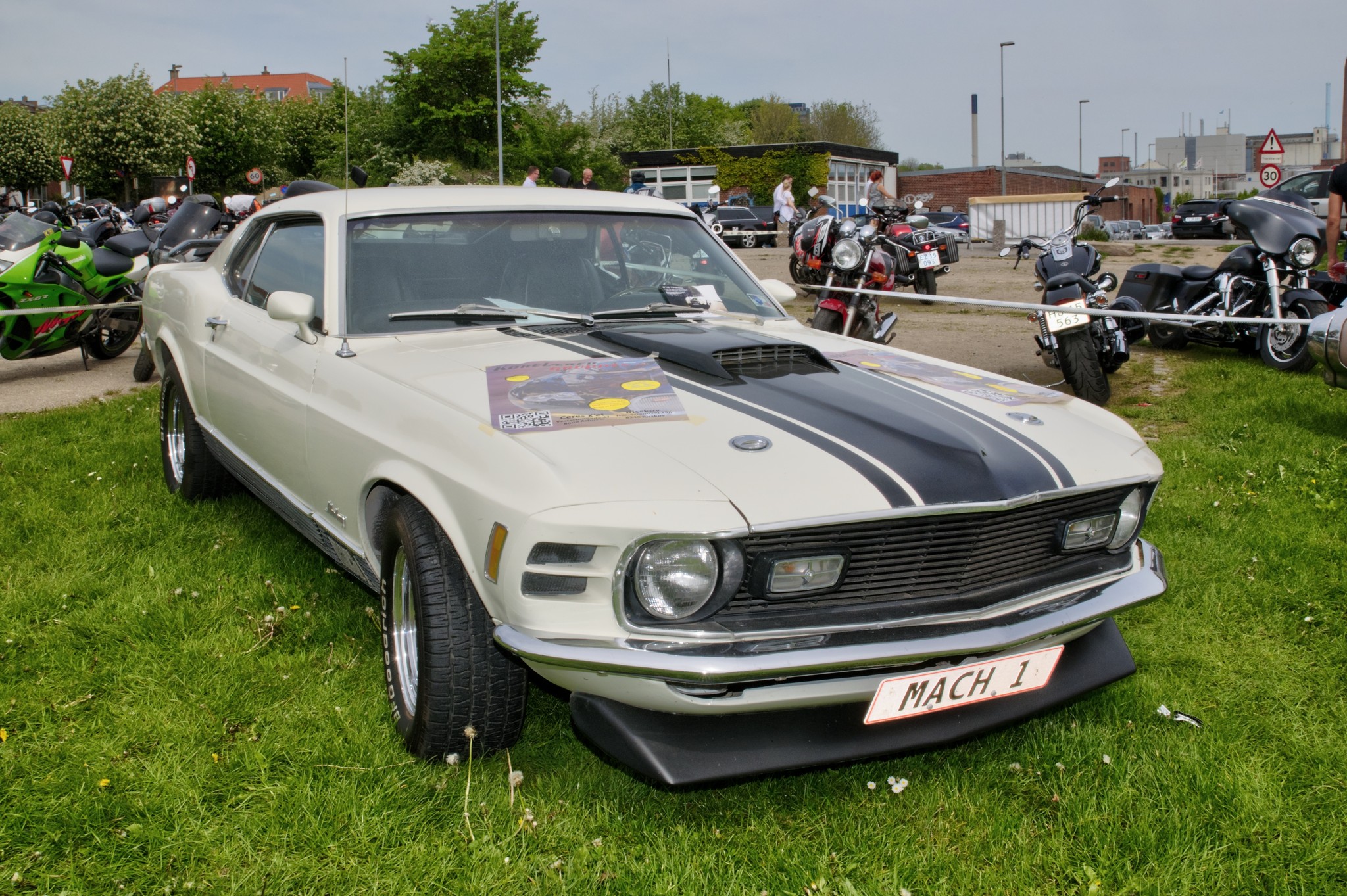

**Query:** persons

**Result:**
xmin=1327 ymin=161 xmax=1347 ymax=280
xmin=865 ymin=169 xmax=899 ymax=228
xmin=522 ymin=165 xmax=665 ymax=201
xmin=773 ymin=175 xmax=800 ymax=246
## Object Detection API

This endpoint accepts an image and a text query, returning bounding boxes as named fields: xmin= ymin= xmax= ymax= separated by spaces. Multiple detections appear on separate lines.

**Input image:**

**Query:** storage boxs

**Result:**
xmin=85 ymin=198 xmax=114 ymax=216
xmin=140 ymin=197 xmax=166 ymax=213
xmin=185 ymin=194 xmax=222 ymax=211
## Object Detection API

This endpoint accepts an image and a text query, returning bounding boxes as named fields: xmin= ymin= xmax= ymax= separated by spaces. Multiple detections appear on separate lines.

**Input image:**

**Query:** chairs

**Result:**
xmin=352 ymin=258 xmax=416 ymax=333
xmin=498 ymin=248 xmax=604 ymax=316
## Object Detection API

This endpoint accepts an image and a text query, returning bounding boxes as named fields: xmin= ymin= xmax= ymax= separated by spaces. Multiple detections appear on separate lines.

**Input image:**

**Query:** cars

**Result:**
xmin=1272 ymin=169 xmax=1347 ymax=233
xmin=135 ymin=189 xmax=1166 ymax=791
xmin=1088 ymin=215 xmax=1175 ymax=240
xmin=910 ymin=212 xmax=970 ymax=236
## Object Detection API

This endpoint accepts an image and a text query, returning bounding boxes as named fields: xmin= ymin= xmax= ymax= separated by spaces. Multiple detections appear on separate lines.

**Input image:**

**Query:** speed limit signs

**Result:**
xmin=247 ymin=169 xmax=262 ymax=184
xmin=1259 ymin=164 xmax=1281 ymax=188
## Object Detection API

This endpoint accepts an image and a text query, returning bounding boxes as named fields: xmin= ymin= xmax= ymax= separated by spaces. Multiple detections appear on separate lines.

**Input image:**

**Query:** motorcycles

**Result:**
xmin=998 ymin=178 xmax=1130 ymax=407
xmin=778 ymin=187 xmax=958 ymax=346
xmin=549 ymin=167 xmax=728 ymax=300
xmin=1111 ymin=189 xmax=1330 ymax=375
xmin=0 ymin=167 xmax=368 ymax=370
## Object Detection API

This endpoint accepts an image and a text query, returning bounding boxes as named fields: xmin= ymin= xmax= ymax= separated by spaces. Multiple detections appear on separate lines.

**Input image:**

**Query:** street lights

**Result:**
xmin=1148 ymin=144 xmax=1155 ymax=225
xmin=1078 ymin=99 xmax=1089 ymax=192
xmin=1191 ymin=164 xmax=1196 ymax=194
xmin=1182 ymin=159 xmax=1187 ymax=204
xmin=1167 ymin=152 xmax=1174 ymax=222
xmin=999 ymin=42 xmax=1014 ymax=195
xmin=1122 ymin=128 xmax=1129 ymax=221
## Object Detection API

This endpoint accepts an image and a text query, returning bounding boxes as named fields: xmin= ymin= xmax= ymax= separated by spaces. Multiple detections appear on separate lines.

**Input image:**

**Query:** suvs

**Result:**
xmin=1172 ymin=198 xmax=1241 ymax=240
xmin=697 ymin=206 xmax=776 ymax=249
